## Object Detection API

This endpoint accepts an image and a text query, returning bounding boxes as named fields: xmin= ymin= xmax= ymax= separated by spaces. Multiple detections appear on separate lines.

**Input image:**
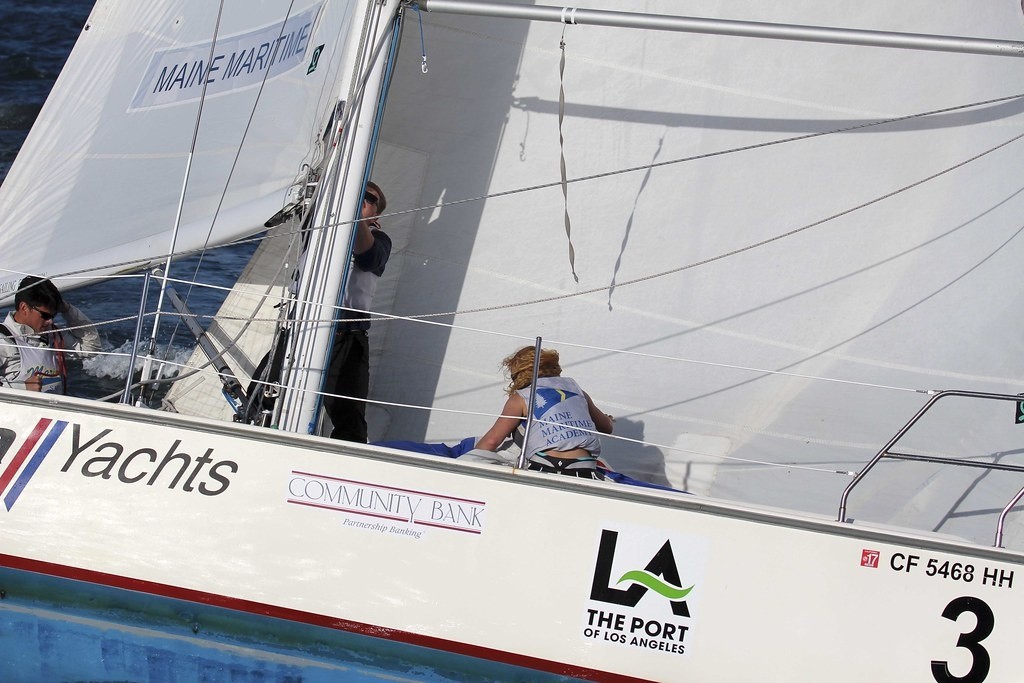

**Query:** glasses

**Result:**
xmin=364 ymin=191 xmax=379 ymax=209
xmin=31 ymin=305 xmax=58 ymax=320
xmin=511 ymin=372 xmax=521 ymax=380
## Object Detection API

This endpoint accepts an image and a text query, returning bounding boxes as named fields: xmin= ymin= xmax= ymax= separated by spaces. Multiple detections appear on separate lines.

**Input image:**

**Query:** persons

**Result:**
xmin=476 ymin=345 xmax=613 ymax=479
xmin=248 ymin=181 xmax=392 ymax=443
xmin=0 ymin=276 xmax=102 ymax=394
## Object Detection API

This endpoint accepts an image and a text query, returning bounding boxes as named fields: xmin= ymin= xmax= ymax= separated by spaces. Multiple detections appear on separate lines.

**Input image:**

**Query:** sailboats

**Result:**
xmin=0 ymin=0 xmax=1024 ymax=683
